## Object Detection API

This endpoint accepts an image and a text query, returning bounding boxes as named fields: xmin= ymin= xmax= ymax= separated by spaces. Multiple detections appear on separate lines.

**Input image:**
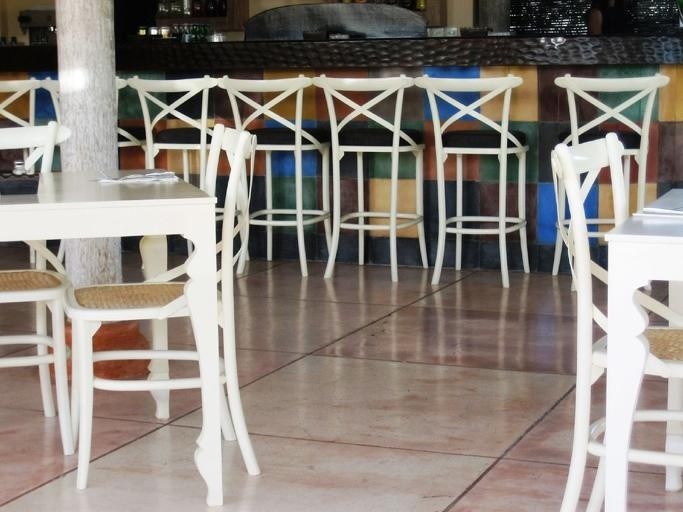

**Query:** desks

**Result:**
xmin=602 ymin=184 xmax=682 ymax=512
xmin=1 ymin=169 xmax=227 ymax=510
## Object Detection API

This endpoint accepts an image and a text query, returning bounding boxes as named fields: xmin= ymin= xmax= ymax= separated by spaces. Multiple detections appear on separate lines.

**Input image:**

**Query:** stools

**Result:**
xmin=1 ymin=119 xmax=77 ymax=460
xmin=59 ymin=123 xmax=265 ymax=491
xmin=312 ymin=72 xmax=430 ymax=284
xmin=549 ymin=72 xmax=671 ymax=277
xmin=415 ymin=73 xmax=533 ymax=290
xmin=40 ymin=70 xmax=148 ymax=169
xmin=1 ymin=74 xmax=41 ymax=194
xmin=129 ymin=70 xmax=226 ymax=263
xmin=551 ymin=132 xmax=683 ymax=512
xmin=216 ymin=71 xmax=332 ymax=279
